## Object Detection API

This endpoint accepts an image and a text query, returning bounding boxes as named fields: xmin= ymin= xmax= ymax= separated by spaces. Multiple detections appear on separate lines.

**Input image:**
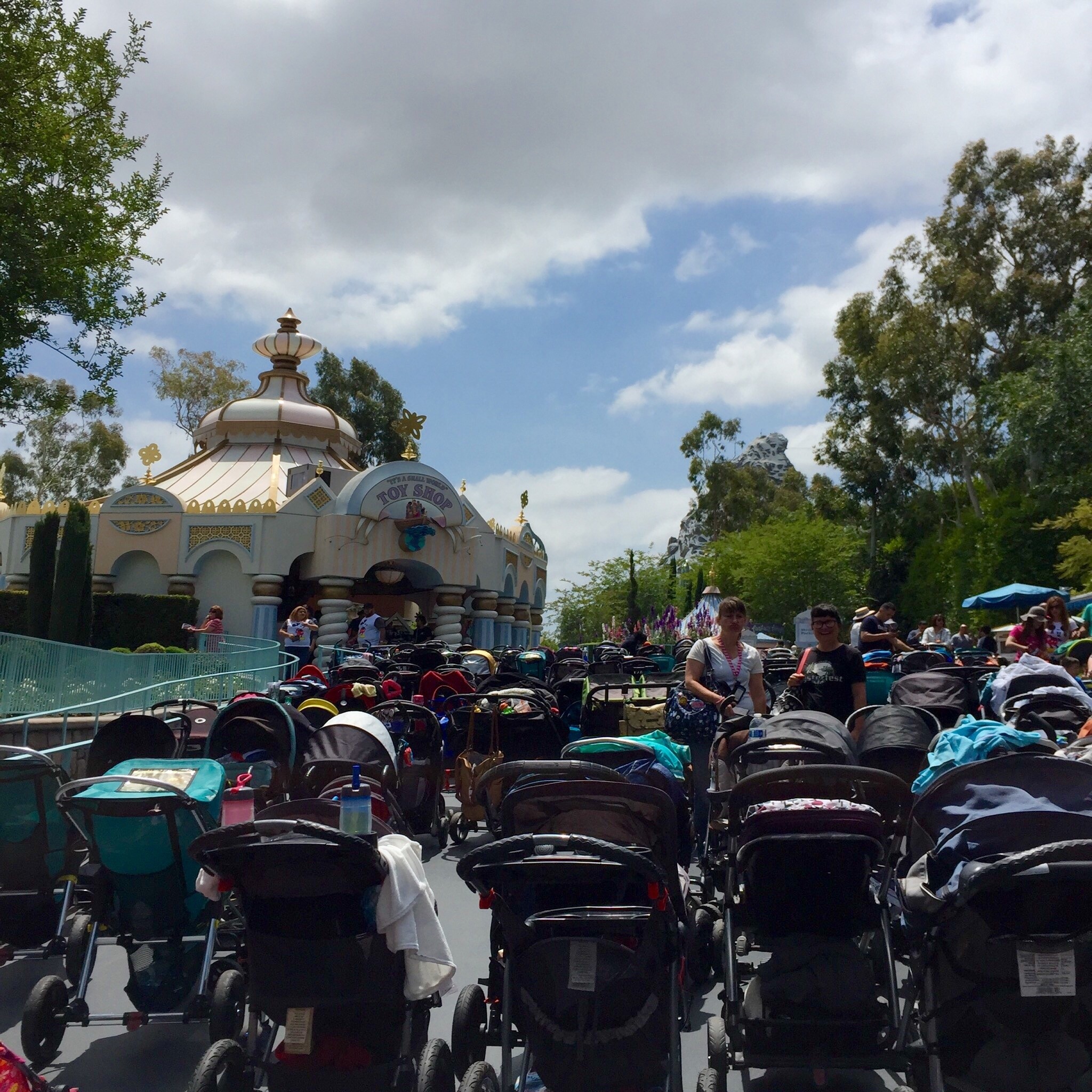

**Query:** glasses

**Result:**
xmin=209 ymin=610 xmax=216 ymax=613
xmin=937 ymin=618 xmax=944 ymax=621
xmin=811 ymin=620 xmax=837 ymax=628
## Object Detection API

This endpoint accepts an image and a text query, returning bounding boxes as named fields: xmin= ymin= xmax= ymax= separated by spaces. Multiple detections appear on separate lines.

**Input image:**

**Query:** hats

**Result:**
xmin=1020 ymin=606 xmax=1049 ymax=622
xmin=362 ymin=603 xmax=373 ymax=609
xmin=853 ymin=607 xmax=875 ymax=620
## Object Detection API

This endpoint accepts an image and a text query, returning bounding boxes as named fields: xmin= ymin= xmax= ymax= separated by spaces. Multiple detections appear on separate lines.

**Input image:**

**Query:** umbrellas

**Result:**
xmin=962 ymin=581 xmax=1071 ymax=626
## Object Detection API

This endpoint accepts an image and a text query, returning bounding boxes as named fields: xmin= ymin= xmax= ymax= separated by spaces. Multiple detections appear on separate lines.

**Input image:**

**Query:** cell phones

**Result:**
xmin=345 ymin=641 xmax=354 ymax=645
xmin=292 ymin=635 xmax=300 ymax=642
xmin=896 ymin=630 xmax=902 ymax=634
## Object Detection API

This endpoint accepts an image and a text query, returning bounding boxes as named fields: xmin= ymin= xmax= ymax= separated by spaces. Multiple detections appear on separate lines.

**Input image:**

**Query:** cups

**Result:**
xmin=267 ymin=682 xmax=282 ymax=703
xmin=514 ymin=700 xmax=532 ymax=713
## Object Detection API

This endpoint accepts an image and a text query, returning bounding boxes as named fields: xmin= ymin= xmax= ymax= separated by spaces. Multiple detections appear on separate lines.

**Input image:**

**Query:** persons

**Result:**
xmin=279 ymin=602 xmax=431 ymax=679
xmin=188 ymin=605 xmax=227 ymax=654
xmin=626 ymin=597 xmax=1092 ymax=864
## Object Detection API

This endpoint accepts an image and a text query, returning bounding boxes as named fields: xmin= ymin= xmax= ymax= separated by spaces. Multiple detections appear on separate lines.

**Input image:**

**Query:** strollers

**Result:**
xmin=0 ymin=638 xmax=1092 ymax=1092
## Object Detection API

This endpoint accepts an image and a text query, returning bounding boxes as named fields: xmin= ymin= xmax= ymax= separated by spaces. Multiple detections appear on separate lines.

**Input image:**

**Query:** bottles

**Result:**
xmin=892 ymin=659 xmax=899 ymax=673
xmin=395 ymin=649 xmax=400 ymax=654
xmin=659 ymin=645 xmax=664 ymax=649
xmin=551 ymin=707 xmax=559 ymax=717
xmin=749 ymin=713 xmax=767 ymax=741
xmin=499 ymin=701 xmax=512 ymax=715
xmin=217 ymin=773 xmax=254 ymax=827
xmin=474 ymin=705 xmax=481 ymax=713
xmin=412 ymin=695 xmax=425 ymax=732
xmin=340 ymin=766 xmax=372 ymax=834
xmin=283 ymin=696 xmax=292 ymax=707
xmin=582 ymin=647 xmax=588 ymax=661
xmin=403 ymin=742 xmax=413 ymax=766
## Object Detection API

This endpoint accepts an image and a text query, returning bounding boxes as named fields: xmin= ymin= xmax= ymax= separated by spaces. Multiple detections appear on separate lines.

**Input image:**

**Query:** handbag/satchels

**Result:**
xmin=770 ymin=684 xmax=804 ymax=715
xmin=664 ymin=677 xmax=720 ymax=742
xmin=455 ymin=692 xmax=504 ymax=821
